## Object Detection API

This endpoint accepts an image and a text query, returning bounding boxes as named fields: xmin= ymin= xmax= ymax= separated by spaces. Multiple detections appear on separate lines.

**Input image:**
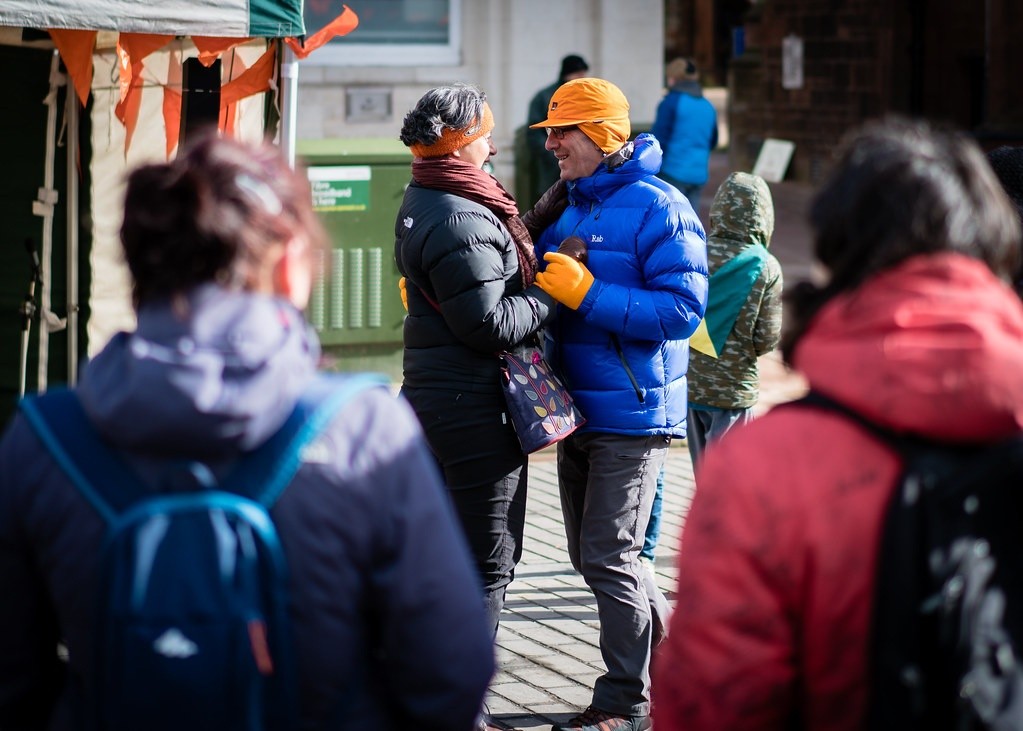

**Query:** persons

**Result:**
xmin=651 ymin=59 xmax=718 ymax=214
xmin=522 ymin=54 xmax=591 ymax=245
xmin=529 ymin=79 xmax=709 ymax=731
xmin=694 ymin=174 xmax=783 ymax=481
xmin=651 ymin=125 xmax=1022 ymax=731
xmin=400 ymin=84 xmax=586 ymax=731
xmin=1 ymin=138 xmax=495 ymax=731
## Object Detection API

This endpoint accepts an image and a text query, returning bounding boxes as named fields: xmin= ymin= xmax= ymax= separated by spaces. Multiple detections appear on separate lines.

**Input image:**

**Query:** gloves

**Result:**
xmin=398 ymin=276 xmax=409 ymax=312
xmin=532 ymin=251 xmax=595 ymax=311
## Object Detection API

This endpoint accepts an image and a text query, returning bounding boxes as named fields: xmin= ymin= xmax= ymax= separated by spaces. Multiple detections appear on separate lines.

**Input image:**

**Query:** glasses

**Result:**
xmin=545 ymin=126 xmax=579 ymax=139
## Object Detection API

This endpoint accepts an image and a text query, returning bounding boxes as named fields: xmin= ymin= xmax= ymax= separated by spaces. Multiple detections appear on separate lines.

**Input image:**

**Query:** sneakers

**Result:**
xmin=552 ymin=704 xmax=652 ymax=731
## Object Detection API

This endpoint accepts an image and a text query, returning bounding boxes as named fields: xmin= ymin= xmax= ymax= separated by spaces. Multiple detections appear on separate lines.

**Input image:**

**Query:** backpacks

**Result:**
xmin=17 ymin=370 xmax=388 ymax=731
xmin=775 ymin=387 xmax=1023 ymax=731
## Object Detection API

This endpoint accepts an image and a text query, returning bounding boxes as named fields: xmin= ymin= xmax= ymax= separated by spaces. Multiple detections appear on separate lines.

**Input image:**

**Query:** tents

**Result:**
xmin=0 ymin=2 xmax=305 ymax=175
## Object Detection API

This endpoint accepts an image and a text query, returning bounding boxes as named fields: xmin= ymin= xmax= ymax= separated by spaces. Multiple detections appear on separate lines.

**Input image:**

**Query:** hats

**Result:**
xmin=561 ymin=55 xmax=588 ymax=74
xmin=529 ymin=77 xmax=631 ymax=154
xmin=667 ymin=57 xmax=700 ymax=82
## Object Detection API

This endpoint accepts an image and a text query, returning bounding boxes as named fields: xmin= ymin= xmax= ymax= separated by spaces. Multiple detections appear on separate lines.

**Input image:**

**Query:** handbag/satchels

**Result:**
xmin=497 ymin=346 xmax=586 ymax=456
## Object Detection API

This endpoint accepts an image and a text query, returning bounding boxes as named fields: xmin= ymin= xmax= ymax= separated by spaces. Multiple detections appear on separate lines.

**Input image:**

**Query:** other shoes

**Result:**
xmin=472 ymin=710 xmax=515 ymax=731
